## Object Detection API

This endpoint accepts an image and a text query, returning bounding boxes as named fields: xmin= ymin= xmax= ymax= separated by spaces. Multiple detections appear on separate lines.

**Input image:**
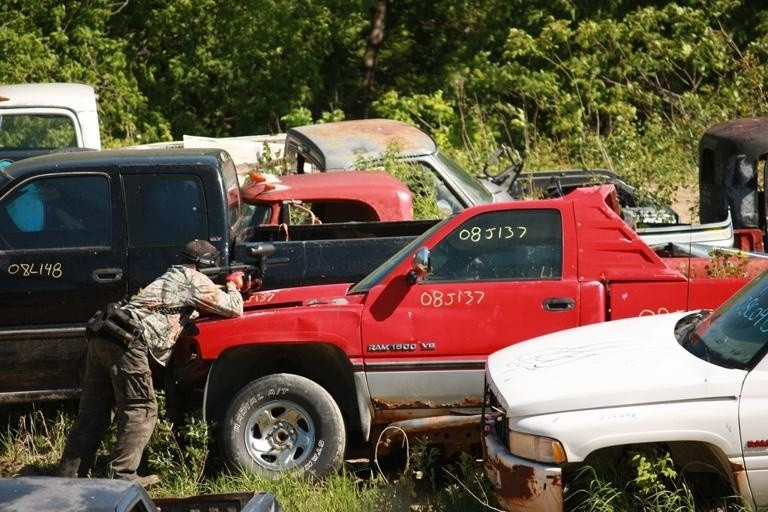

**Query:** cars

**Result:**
xmin=476 ymin=265 xmax=768 ymax=512
xmin=160 ymin=186 xmax=768 ymax=488
xmin=0 ymin=475 xmax=281 ymax=512
xmin=225 ymin=116 xmax=743 ymax=253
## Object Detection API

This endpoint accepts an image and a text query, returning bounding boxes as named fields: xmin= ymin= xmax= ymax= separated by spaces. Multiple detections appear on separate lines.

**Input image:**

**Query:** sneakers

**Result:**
xmin=115 ymin=470 xmax=161 ymax=492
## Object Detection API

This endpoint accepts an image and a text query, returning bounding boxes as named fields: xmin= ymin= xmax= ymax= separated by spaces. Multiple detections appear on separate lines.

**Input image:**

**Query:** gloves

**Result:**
xmin=225 ymin=270 xmax=263 ymax=293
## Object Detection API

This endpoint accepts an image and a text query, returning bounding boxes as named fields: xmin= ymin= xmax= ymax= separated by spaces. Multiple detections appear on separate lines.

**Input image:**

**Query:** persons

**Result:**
xmin=58 ymin=237 xmax=264 ymax=489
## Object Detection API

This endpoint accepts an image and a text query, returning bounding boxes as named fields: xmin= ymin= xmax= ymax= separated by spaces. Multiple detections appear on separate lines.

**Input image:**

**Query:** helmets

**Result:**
xmin=180 ymin=240 xmax=220 ymax=280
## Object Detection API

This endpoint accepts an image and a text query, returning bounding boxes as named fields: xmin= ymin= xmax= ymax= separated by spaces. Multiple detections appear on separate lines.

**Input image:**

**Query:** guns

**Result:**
xmin=198 ymin=244 xmax=290 ymax=282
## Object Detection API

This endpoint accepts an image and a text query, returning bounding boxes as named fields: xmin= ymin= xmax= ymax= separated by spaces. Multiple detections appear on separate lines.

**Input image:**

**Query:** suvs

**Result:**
xmin=698 ymin=118 xmax=768 ymax=253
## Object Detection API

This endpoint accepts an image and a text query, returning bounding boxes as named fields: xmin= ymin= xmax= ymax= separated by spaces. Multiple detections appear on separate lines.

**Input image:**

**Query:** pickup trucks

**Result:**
xmin=0 ymin=82 xmax=297 ymax=195
xmin=0 ymin=147 xmax=531 ymax=403
